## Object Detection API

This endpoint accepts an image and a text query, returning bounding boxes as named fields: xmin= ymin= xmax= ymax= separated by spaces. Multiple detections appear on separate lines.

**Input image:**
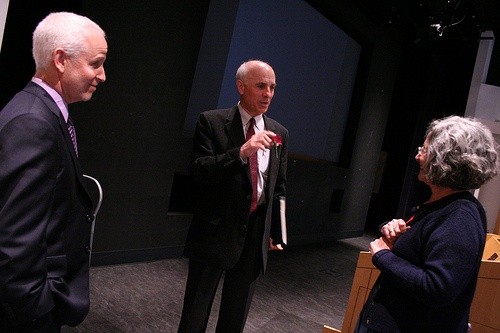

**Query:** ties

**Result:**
xmin=246 ymin=117 xmax=258 ymax=212
xmin=66 ymin=115 xmax=78 ymax=158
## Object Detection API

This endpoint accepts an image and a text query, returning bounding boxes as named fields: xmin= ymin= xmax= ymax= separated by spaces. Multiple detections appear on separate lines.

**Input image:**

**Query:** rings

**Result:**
xmin=389 ymin=230 xmax=394 ymax=232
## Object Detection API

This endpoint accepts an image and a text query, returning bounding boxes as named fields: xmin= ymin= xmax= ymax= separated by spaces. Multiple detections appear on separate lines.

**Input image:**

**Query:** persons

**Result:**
xmin=0 ymin=13 xmax=108 ymax=333
xmin=352 ymin=115 xmax=499 ymax=333
xmin=177 ymin=59 xmax=290 ymax=332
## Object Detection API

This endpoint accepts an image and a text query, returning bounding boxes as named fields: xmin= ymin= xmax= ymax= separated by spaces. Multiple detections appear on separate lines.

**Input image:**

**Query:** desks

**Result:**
xmin=340 ymin=232 xmax=500 ymax=333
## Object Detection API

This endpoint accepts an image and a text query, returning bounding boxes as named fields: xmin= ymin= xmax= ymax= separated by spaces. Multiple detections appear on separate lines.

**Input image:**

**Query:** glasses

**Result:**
xmin=417 ymin=146 xmax=428 ymax=158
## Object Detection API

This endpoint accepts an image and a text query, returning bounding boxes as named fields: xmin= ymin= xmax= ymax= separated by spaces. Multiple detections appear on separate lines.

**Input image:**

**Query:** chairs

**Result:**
xmin=82 ymin=174 xmax=104 ymax=272
